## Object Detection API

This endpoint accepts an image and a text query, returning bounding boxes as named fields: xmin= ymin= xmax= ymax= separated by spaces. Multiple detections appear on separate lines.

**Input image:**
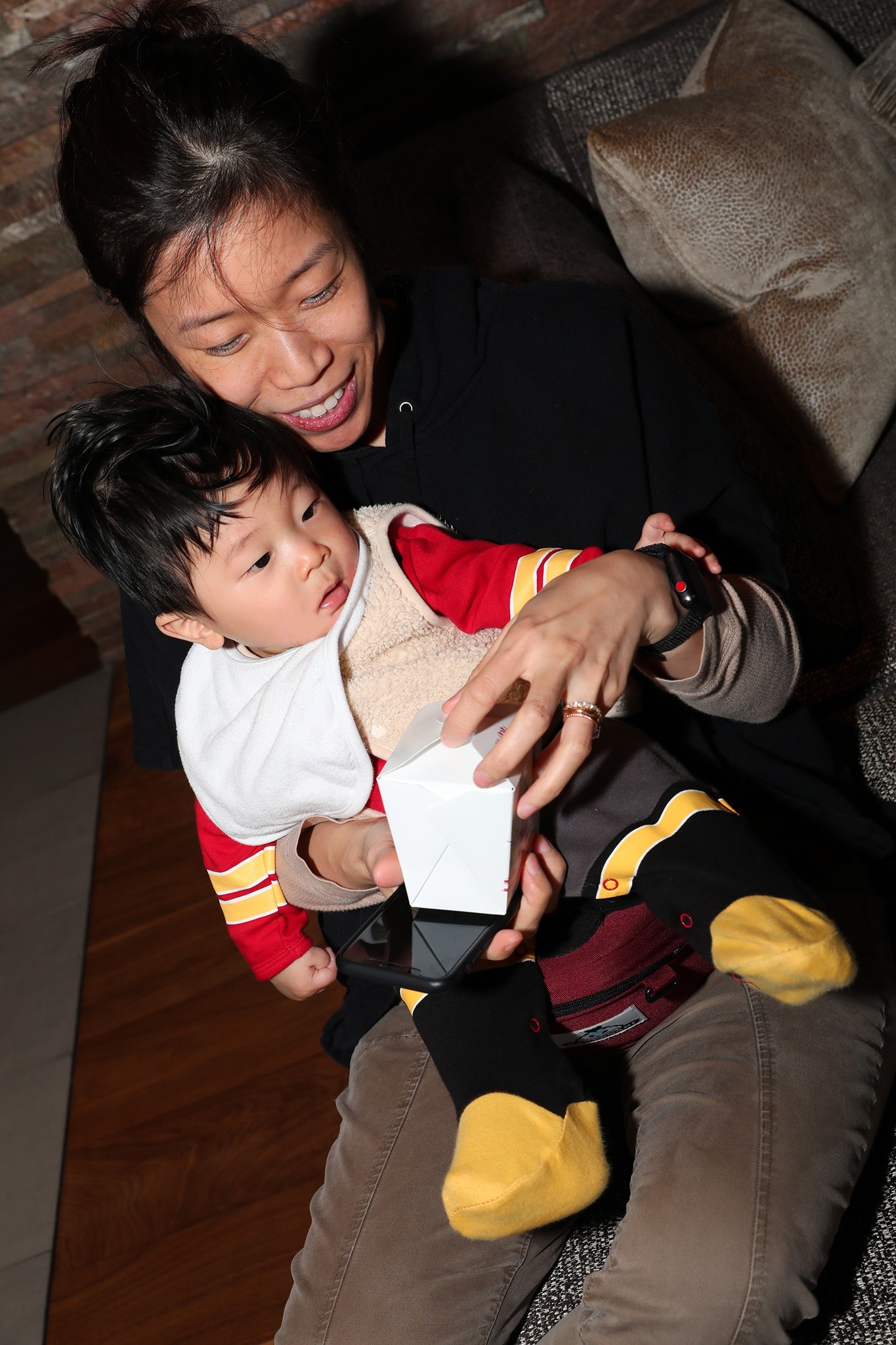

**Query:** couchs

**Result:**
xmin=342 ymin=0 xmax=896 ymax=1345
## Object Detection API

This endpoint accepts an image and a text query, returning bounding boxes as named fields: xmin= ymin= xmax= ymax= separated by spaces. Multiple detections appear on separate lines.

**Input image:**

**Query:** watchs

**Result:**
xmin=636 ymin=543 xmax=714 ymax=654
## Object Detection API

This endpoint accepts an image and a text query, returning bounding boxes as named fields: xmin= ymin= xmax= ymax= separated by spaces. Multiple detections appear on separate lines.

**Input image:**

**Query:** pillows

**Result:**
xmin=582 ymin=0 xmax=896 ymax=489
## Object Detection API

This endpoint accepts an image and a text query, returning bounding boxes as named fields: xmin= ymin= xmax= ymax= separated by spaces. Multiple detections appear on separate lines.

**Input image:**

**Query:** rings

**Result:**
xmin=563 ymin=701 xmax=602 ymax=739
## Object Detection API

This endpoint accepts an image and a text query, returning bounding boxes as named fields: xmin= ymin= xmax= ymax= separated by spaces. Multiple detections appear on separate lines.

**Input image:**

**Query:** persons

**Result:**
xmin=45 ymin=341 xmax=858 ymax=1242
xmin=32 ymin=1 xmax=896 ymax=1345
